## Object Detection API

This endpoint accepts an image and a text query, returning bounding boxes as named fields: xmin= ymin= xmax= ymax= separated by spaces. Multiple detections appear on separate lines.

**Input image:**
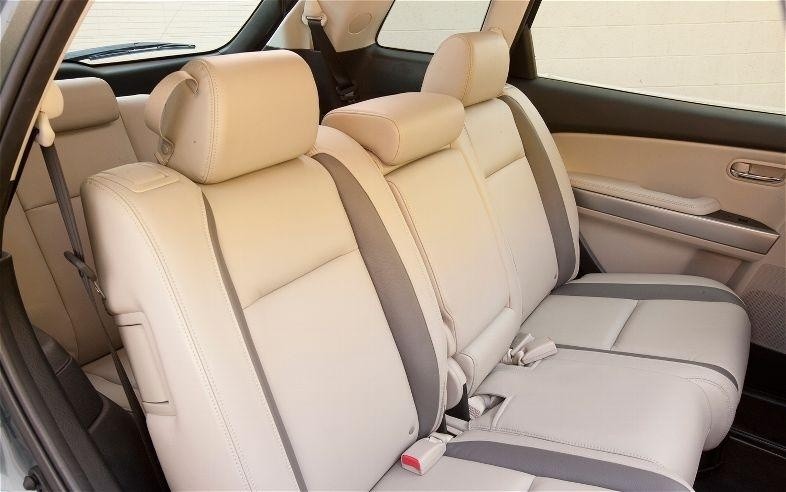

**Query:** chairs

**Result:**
xmin=111 ymin=94 xmax=165 ymax=163
xmin=2 ymin=68 xmax=163 ymax=423
xmin=319 ymin=93 xmax=710 ymax=491
xmin=79 ymin=44 xmax=701 ymax=490
xmin=419 ymin=25 xmax=780 ymax=456
